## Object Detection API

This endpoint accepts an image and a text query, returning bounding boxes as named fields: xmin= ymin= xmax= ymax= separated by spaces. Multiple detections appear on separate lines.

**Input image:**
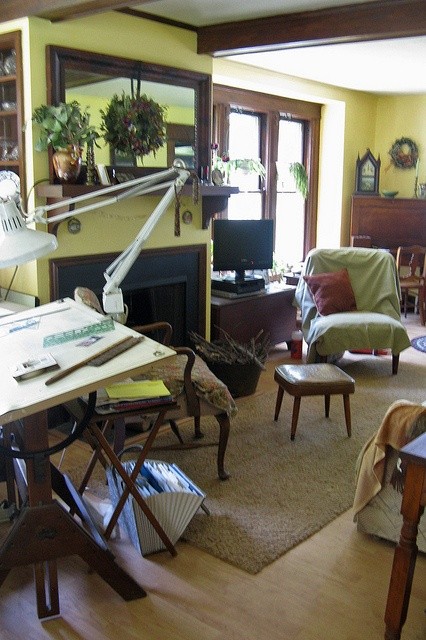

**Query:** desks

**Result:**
xmin=210 ymin=284 xmax=298 ymax=351
xmin=0 ymin=294 xmax=177 ymax=620
xmin=282 ymin=272 xmax=300 ymax=285
xmin=0 ymin=287 xmax=38 ymax=319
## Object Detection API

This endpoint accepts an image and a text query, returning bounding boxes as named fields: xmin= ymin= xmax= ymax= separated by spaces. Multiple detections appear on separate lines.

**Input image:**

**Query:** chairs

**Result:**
xmin=74 ymin=286 xmax=239 ymax=481
xmin=383 ymin=433 xmax=426 ymax=640
xmin=395 ymin=244 xmax=426 ymax=326
xmin=291 ymin=245 xmax=411 ymax=375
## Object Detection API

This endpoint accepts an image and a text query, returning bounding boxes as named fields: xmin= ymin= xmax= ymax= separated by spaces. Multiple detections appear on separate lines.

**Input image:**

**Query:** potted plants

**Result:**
xmin=32 ymin=100 xmax=102 ymax=183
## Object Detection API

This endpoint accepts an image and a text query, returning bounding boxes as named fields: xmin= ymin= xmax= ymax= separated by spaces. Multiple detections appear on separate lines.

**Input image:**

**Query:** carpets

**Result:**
xmin=411 ymin=335 xmax=426 ymax=353
xmin=64 ymin=354 xmax=426 ymax=576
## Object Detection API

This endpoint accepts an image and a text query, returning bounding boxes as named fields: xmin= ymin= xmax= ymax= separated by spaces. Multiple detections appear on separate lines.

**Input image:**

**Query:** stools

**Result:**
xmin=273 ymin=362 xmax=356 ymax=442
xmin=62 ymin=396 xmax=180 ymax=557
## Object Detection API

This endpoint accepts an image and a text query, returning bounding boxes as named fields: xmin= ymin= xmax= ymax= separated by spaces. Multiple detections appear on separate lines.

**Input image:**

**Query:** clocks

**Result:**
xmin=355 ymin=147 xmax=381 ymax=195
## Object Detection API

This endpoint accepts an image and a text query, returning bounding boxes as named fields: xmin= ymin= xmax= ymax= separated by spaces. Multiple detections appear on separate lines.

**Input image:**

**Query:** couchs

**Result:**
xmin=352 ymin=399 xmax=426 ymax=554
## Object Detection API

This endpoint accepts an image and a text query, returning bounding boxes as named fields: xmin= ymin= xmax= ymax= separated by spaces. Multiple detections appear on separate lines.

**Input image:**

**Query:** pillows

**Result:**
xmin=303 ymin=268 xmax=356 ymax=316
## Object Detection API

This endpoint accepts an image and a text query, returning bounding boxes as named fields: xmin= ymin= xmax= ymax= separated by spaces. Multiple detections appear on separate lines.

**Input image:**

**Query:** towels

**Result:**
xmin=352 ymin=398 xmax=426 ymax=524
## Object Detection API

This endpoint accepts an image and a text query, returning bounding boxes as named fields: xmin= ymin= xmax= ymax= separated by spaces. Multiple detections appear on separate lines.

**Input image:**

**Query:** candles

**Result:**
xmin=414 ymin=157 xmax=420 ymax=198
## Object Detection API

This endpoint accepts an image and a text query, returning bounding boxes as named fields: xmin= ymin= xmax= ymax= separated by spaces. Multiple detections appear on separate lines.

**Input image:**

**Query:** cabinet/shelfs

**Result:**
xmin=0 ymin=30 xmax=27 ymax=216
xmin=201 ymin=183 xmax=239 ymax=229
xmin=349 ymin=195 xmax=425 ymax=255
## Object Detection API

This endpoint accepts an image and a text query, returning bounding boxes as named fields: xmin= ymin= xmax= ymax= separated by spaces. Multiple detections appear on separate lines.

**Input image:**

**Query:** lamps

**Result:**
xmin=1 ymin=163 xmax=189 ymax=323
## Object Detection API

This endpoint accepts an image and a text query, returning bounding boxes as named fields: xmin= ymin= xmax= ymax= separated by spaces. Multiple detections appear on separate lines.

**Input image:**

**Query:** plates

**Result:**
xmin=0 ymin=170 xmax=21 ymax=206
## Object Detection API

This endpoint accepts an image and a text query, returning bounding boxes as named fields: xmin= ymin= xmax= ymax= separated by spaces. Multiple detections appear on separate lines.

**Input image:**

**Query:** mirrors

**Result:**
xmin=43 ymin=44 xmax=212 ymax=189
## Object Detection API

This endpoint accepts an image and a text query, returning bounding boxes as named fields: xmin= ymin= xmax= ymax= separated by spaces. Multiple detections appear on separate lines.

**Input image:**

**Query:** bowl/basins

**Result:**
xmin=382 ymin=191 xmax=400 ymax=197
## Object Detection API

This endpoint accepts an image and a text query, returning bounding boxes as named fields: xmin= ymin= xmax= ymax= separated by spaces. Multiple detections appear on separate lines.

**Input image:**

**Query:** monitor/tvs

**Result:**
xmin=213 ymin=220 xmax=273 ymax=285
